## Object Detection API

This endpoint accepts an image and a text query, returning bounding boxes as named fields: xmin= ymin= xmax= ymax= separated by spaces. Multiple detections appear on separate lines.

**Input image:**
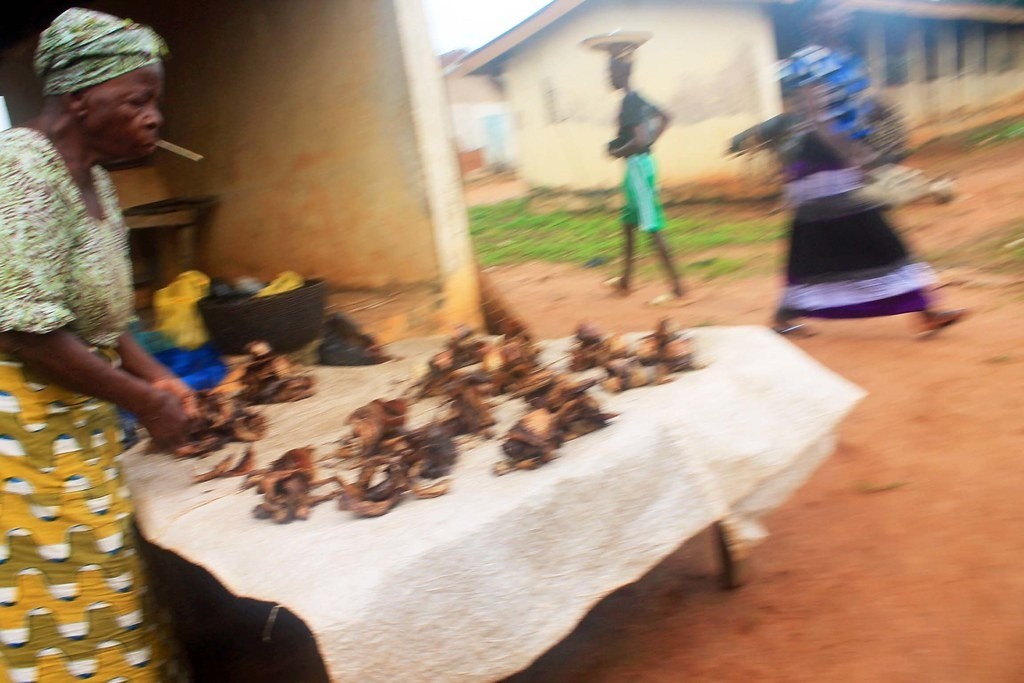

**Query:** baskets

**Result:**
xmin=196 ymin=276 xmax=326 ymax=359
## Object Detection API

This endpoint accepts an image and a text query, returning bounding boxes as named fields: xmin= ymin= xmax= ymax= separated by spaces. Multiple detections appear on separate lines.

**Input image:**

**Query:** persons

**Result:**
xmin=766 ymin=85 xmax=970 ymax=341
xmin=602 ymin=59 xmax=685 ymax=306
xmin=0 ymin=6 xmax=210 ymax=683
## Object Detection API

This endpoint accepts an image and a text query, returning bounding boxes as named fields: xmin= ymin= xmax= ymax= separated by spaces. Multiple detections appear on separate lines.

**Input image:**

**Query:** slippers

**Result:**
xmin=922 ymin=303 xmax=966 ymax=331
xmin=771 ymin=318 xmax=817 ymax=338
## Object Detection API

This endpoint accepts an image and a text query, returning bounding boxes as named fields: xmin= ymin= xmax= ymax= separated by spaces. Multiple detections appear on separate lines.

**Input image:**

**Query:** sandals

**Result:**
xmin=603 ymin=275 xmax=696 ymax=303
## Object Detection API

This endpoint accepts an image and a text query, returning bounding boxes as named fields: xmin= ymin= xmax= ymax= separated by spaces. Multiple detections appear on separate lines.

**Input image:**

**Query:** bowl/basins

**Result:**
xmin=198 ymin=277 xmax=330 ymax=355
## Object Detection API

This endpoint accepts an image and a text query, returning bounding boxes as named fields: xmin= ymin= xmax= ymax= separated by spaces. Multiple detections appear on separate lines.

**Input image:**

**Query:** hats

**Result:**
xmin=34 ymin=7 xmax=169 ymax=96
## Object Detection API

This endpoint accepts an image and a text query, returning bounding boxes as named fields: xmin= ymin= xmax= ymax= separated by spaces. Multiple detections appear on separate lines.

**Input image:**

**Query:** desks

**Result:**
xmin=118 ymin=312 xmax=873 ymax=675
